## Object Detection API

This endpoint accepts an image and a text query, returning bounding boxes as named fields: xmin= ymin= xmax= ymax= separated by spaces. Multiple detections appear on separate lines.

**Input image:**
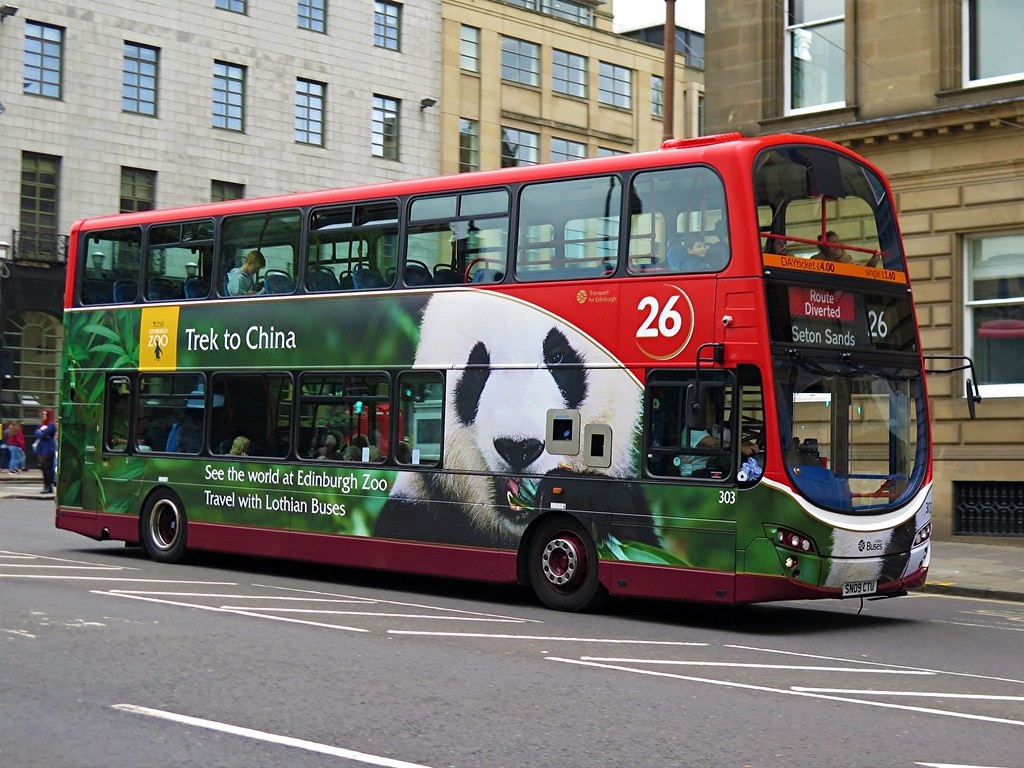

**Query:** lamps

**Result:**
xmin=421 ymin=98 xmax=436 ymax=110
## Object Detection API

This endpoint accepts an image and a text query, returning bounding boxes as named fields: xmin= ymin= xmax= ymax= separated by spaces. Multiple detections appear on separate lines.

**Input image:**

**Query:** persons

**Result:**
xmin=762 ymin=231 xmax=793 ymax=256
xmin=0 ymin=420 xmax=28 ymax=474
xmin=678 ymin=232 xmax=711 ymax=272
xmin=33 ymin=408 xmax=57 ymax=494
xmin=52 ymin=420 xmax=60 ymax=487
xmin=221 ymin=250 xmax=265 ymax=296
xmin=396 ymin=440 xmax=412 ymax=464
xmin=316 ymin=430 xmax=383 ymax=462
xmin=809 ymin=231 xmax=854 ymax=263
xmin=704 ymin=219 xmax=730 ymax=271
xmin=110 ymin=411 xmax=152 ymax=452
xmin=230 ymin=436 xmax=251 ymax=457
xmin=679 ymin=401 xmax=759 ymax=478
xmin=164 ymin=407 xmax=200 ymax=454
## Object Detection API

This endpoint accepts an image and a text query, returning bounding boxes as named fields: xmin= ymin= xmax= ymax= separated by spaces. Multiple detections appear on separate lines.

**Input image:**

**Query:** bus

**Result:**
xmin=54 ymin=133 xmax=982 ymax=612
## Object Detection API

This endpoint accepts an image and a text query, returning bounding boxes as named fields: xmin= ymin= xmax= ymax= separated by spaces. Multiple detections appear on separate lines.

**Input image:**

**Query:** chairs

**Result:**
xmin=82 ymin=238 xmax=686 ymax=308
xmin=648 ymin=411 xmax=678 ymax=475
xmin=218 ymin=440 xmax=233 ymax=455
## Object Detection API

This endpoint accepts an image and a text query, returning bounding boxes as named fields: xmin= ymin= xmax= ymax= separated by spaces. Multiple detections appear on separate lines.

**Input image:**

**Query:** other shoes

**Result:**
xmin=9 ymin=471 xmax=15 ymax=474
xmin=21 ymin=467 xmax=28 ymax=471
xmin=13 ymin=469 xmax=20 ymax=474
xmin=40 ymin=488 xmax=53 ymax=494
